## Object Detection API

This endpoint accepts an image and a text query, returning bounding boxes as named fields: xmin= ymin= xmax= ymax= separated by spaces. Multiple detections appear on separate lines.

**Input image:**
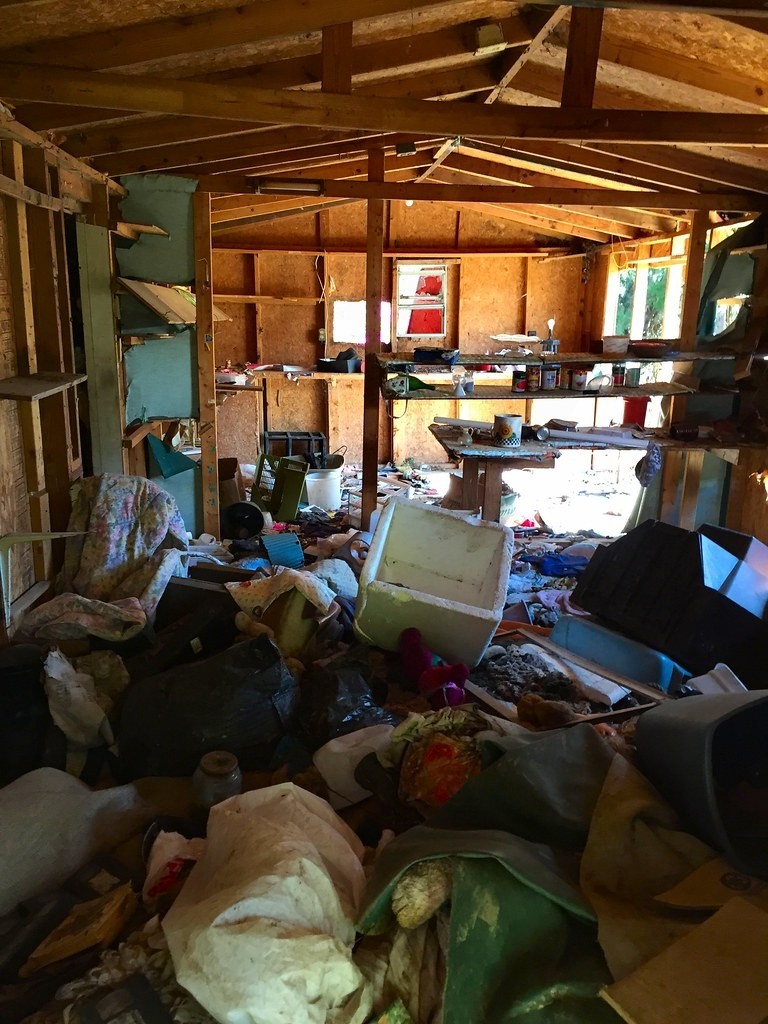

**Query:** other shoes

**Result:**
xmin=335 ymin=347 xmax=358 ymax=362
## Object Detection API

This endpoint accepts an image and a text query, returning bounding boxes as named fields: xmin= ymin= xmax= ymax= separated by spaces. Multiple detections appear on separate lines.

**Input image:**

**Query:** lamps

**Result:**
xmin=394 ymin=145 xmax=417 ymax=157
xmin=466 ymin=18 xmax=510 ymax=56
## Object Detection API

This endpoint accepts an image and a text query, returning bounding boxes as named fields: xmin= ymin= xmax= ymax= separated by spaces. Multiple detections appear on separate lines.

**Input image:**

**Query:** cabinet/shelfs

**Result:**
xmin=376 ymin=352 xmax=736 ymax=400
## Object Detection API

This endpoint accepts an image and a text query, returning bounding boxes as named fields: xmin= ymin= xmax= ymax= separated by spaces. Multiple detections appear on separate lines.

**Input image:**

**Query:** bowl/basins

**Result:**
xmin=630 ymin=340 xmax=672 ymax=358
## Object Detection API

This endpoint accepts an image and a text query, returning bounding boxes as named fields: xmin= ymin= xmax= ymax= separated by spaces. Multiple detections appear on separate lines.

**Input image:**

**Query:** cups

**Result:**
xmin=512 ymin=365 xmax=587 ymax=393
xmin=611 ymin=366 xmax=641 ymax=388
xmin=603 ymin=336 xmax=629 ymax=358
xmin=493 ymin=414 xmax=523 ymax=448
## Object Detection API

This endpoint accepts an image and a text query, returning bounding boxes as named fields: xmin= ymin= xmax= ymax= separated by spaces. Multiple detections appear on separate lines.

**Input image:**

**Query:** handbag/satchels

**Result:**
xmin=381 ymin=376 xmax=409 ymax=399
xmin=414 ymin=346 xmax=460 ymax=365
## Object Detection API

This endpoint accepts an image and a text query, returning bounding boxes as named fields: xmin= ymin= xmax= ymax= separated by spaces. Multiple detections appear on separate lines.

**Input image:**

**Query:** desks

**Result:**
xmin=423 ymin=423 xmax=741 ymax=532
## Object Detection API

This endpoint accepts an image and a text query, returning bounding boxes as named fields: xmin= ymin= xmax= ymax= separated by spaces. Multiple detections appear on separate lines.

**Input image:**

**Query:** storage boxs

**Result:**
xmin=346 ymin=495 xmax=516 ymax=667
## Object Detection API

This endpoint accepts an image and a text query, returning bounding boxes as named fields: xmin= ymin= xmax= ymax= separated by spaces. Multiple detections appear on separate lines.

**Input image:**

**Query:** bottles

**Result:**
xmin=193 ymin=750 xmax=242 ymax=817
